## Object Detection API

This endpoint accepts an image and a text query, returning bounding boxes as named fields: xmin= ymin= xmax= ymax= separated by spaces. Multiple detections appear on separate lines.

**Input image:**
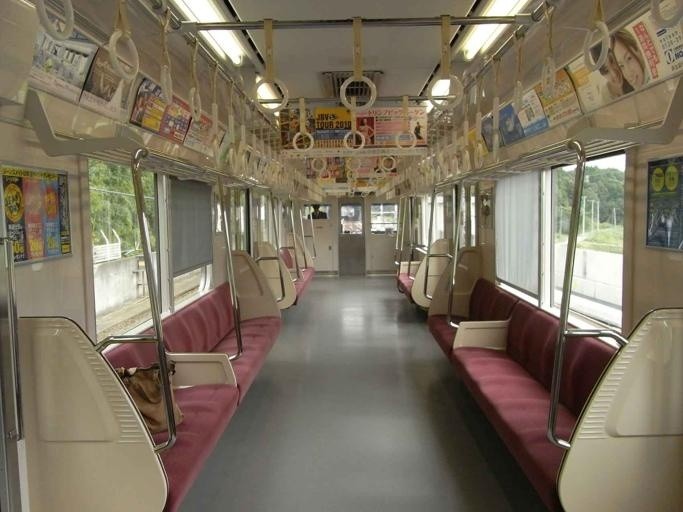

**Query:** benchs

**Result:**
xmin=396 ymin=248 xmax=624 ymax=512
xmin=93 ymin=245 xmax=315 ymax=512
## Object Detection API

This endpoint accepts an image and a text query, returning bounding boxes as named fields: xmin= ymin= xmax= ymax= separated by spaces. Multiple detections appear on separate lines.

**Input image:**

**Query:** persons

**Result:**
xmin=308 ymin=205 xmax=327 ymax=219
xmin=357 ymin=119 xmax=374 ymax=145
xmin=590 ymin=27 xmax=649 ymax=106
xmin=413 ymin=121 xmax=423 ymax=140
xmin=130 ymin=94 xmax=144 ymax=122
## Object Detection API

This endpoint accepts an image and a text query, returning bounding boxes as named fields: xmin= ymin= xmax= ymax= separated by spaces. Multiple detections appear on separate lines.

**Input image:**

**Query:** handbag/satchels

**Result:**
xmin=114 ymin=360 xmax=183 ymax=436
